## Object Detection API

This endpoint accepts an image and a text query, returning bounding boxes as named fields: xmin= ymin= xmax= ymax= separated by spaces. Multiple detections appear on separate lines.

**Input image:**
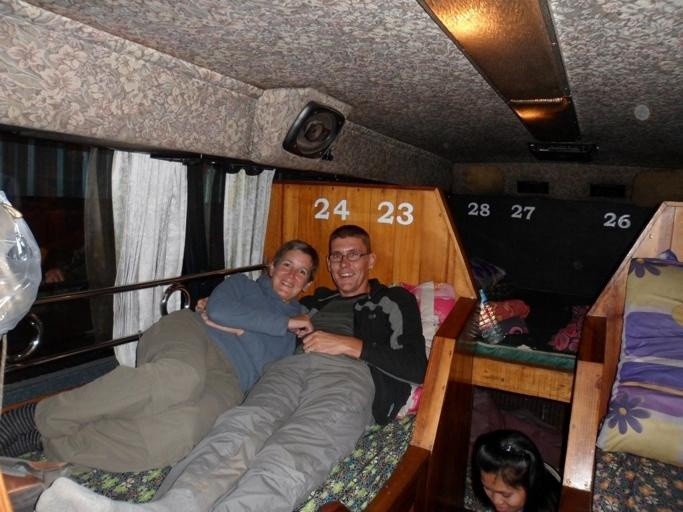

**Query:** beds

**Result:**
xmin=1 ymin=180 xmax=483 ymax=512
xmin=560 ymin=200 xmax=683 ymax=511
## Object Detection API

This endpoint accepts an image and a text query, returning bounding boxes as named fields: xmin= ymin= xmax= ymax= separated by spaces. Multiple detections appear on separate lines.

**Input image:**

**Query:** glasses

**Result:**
xmin=328 ymin=252 xmax=369 ymax=262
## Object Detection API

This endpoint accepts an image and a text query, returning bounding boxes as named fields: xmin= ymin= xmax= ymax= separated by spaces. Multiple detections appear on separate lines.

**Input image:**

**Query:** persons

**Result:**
xmin=1 ymin=240 xmax=320 ymax=470
xmin=36 ymin=225 xmax=427 ymax=512
xmin=468 ymin=428 xmax=562 ymax=511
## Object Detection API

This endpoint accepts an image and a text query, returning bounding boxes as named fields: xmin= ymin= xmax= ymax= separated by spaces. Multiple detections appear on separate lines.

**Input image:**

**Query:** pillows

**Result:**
xmin=594 ymin=250 xmax=683 ymax=469
